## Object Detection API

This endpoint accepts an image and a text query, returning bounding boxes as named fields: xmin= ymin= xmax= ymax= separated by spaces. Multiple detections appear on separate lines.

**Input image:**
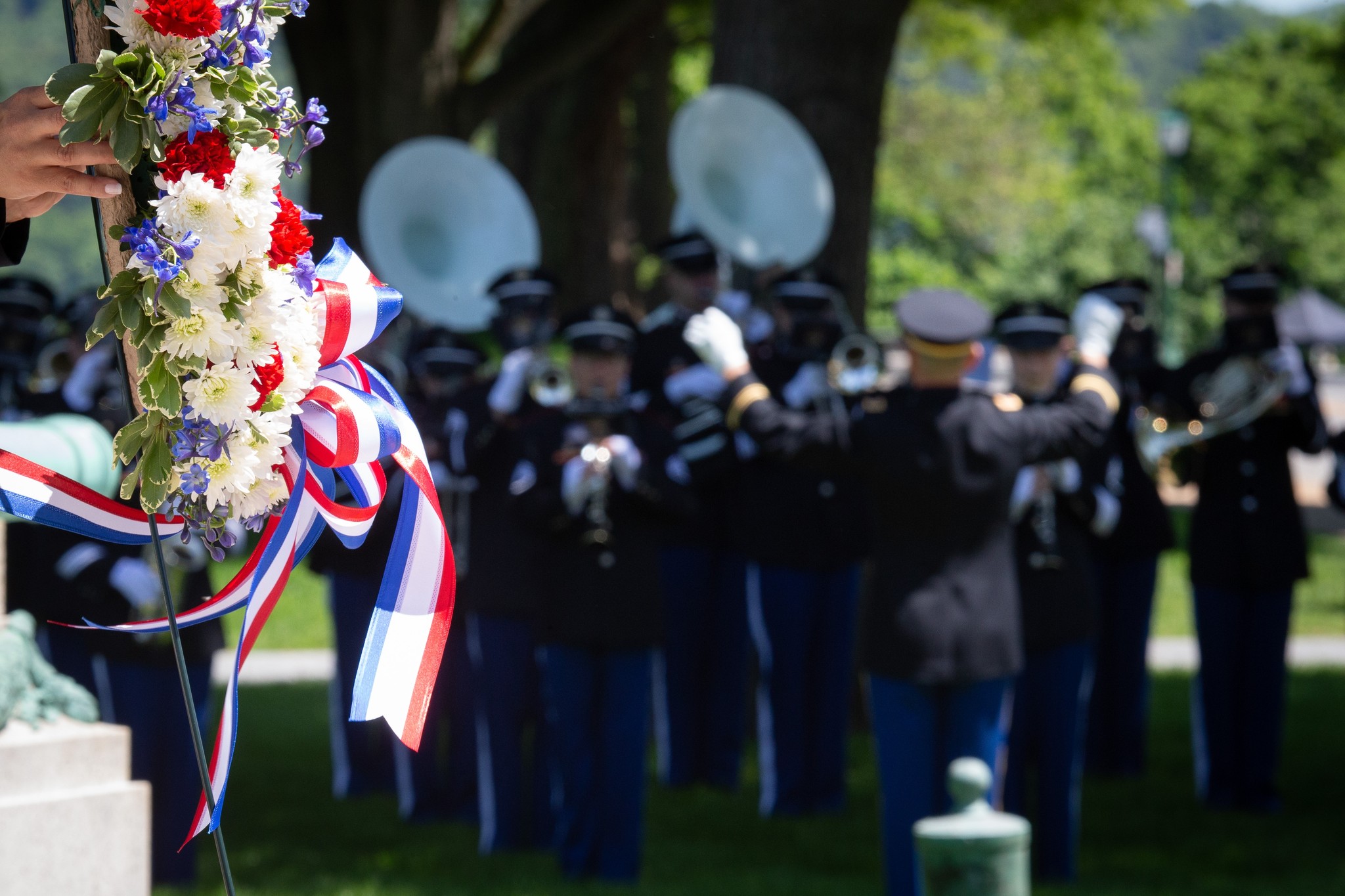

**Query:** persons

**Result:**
xmin=681 ymin=283 xmax=1126 ymax=896
xmin=0 ymin=79 xmax=142 ymax=267
xmin=1 ymin=239 xmax=1345 ymax=876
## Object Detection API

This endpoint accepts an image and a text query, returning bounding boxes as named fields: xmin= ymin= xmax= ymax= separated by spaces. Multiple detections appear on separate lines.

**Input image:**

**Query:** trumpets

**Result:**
xmin=524 ymin=355 xmax=576 ymax=409
xmin=825 ymin=332 xmax=890 ymax=397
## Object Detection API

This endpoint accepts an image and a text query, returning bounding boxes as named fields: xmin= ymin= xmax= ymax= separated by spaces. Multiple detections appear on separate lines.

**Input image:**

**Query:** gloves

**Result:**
xmin=1069 ymin=294 xmax=1126 ymax=359
xmin=681 ymin=305 xmax=749 ymax=369
xmin=603 ymin=435 xmax=641 ymax=493
xmin=664 ymin=363 xmax=727 ymax=405
xmin=784 ymin=362 xmax=827 ymax=409
xmin=489 ymin=347 xmax=532 ymax=410
xmin=559 ymin=454 xmax=591 ymax=514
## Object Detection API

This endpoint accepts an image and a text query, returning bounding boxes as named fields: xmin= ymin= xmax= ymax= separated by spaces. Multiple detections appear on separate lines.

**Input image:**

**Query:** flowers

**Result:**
xmin=45 ymin=0 xmax=327 ymax=563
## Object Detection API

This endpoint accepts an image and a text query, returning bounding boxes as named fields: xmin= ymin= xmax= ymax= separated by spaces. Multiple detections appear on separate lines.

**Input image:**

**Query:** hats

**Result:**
xmin=657 ymin=231 xmax=721 ymax=275
xmin=897 ymin=285 xmax=991 ymax=354
xmin=557 ymin=306 xmax=638 ymax=353
xmin=420 ymin=330 xmax=489 ymax=376
xmin=489 ymin=268 xmax=558 ymax=307
xmin=1220 ymin=268 xmax=1284 ymax=299
xmin=995 ymin=301 xmax=1072 ymax=351
xmin=767 ymin=263 xmax=836 ymax=306
xmin=1082 ymin=275 xmax=1151 ymax=311
